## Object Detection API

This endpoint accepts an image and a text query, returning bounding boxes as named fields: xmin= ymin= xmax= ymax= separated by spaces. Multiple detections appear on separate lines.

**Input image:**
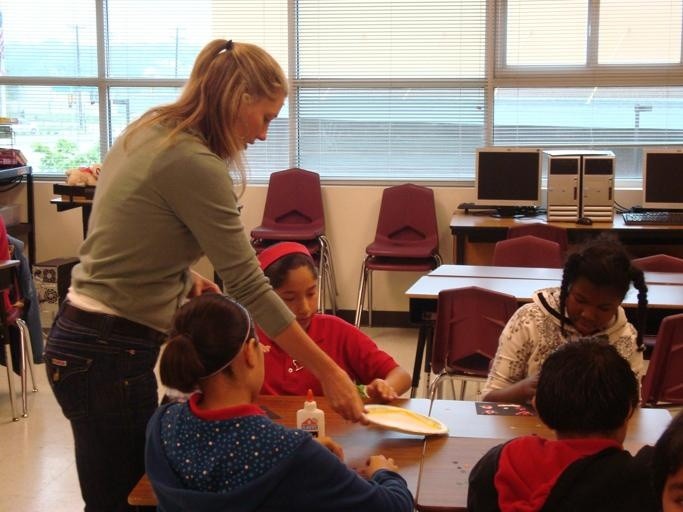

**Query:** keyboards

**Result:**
xmin=622 ymin=211 xmax=683 ymax=226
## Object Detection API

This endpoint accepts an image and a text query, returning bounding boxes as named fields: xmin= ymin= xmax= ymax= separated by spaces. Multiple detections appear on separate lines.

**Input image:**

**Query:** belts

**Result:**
xmin=62 ymin=304 xmax=159 ymax=341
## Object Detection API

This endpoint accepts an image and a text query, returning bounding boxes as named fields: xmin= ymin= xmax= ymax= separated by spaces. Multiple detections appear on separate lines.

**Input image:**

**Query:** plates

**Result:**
xmin=361 ymin=405 xmax=446 ymax=438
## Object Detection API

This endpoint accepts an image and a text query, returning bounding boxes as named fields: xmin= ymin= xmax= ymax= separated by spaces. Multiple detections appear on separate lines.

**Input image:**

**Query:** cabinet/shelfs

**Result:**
xmin=0 ymin=164 xmax=35 ymax=270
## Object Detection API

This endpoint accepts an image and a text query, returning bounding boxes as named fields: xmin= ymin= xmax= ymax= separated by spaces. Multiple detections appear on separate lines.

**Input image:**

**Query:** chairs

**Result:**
xmin=425 ymin=287 xmax=519 ymax=399
xmin=506 ymin=221 xmax=567 ymax=265
xmin=641 ymin=314 xmax=681 ymax=408
xmin=491 ymin=235 xmax=561 ymax=269
xmin=630 ymin=254 xmax=682 ymax=272
xmin=0 ymin=212 xmax=37 ymax=421
xmin=351 ymin=182 xmax=442 ymax=328
xmin=247 ymin=165 xmax=339 ymax=315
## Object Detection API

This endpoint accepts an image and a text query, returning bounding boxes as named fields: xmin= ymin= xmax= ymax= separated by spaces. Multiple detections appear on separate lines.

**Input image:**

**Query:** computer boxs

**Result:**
xmin=543 ymin=149 xmax=581 ymax=223
xmin=581 ymin=150 xmax=613 ymax=223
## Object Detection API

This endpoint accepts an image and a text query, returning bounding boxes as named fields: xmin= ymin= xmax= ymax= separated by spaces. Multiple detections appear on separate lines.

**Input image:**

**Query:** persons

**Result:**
xmin=647 ymin=412 xmax=683 ymax=512
xmin=142 ymin=290 xmax=417 ymax=511
xmin=43 ymin=35 xmax=372 ymax=510
xmin=465 ymin=338 xmax=660 ymax=512
xmin=230 ymin=241 xmax=414 ymax=408
xmin=481 ymin=244 xmax=649 ymax=406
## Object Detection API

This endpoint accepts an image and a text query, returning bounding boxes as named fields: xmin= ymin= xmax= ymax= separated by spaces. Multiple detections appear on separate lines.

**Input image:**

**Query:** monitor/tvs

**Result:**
xmin=475 ymin=147 xmax=543 ymax=218
xmin=641 ymin=149 xmax=683 ymax=210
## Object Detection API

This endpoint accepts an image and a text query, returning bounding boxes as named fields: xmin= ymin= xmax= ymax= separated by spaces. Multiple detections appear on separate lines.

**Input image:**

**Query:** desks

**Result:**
xmin=450 ymin=205 xmax=682 ymax=265
xmin=405 ymin=264 xmax=683 ymax=399
xmin=51 ymin=179 xmax=244 ymax=294
xmin=125 ymin=394 xmax=680 ymax=510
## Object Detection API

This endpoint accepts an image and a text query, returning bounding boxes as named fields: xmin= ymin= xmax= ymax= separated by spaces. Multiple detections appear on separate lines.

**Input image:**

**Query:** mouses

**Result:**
xmin=576 ymin=218 xmax=592 ymax=225
